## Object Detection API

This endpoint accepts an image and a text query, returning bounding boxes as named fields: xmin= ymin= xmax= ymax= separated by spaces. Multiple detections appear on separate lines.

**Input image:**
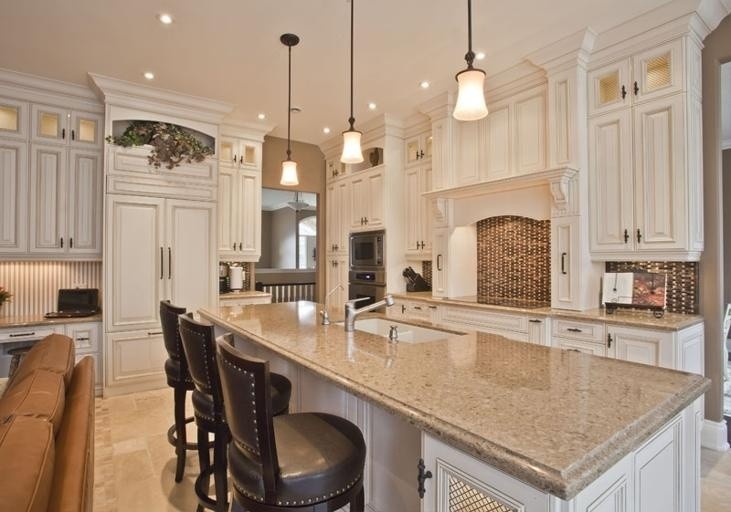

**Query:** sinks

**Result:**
xmin=385 ymin=323 xmax=468 ymax=345
xmin=332 ymin=313 xmax=414 ymax=338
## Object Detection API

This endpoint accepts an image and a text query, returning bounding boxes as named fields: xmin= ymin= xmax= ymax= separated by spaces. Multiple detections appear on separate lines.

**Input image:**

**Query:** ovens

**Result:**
xmin=348 ymin=231 xmax=386 ymax=314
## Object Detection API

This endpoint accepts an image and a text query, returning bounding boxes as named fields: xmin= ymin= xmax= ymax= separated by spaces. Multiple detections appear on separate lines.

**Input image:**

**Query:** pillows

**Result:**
xmin=0 ymin=333 xmax=76 ymax=512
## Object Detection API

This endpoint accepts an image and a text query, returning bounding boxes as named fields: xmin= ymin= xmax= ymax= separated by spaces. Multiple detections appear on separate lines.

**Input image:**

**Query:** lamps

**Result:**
xmin=340 ymin=0 xmax=364 ymax=164
xmin=453 ymin=0 xmax=488 ymax=121
xmin=279 ymin=34 xmax=299 ymax=186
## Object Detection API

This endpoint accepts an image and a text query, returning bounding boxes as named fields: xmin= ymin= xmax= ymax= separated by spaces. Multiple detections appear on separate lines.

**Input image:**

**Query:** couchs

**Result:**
xmin=0 ymin=355 xmax=95 ymax=512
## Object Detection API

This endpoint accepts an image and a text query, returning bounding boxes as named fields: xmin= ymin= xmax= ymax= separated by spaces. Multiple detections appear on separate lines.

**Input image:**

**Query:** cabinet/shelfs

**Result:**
xmin=0 ymin=322 xmax=103 ymax=396
xmin=551 ymin=216 xmax=585 ymax=312
xmin=386 ymin=298 xmax=677 ymax=369
xmin=417 ymin=430 xmax=549 ymax=512
xmin=432 ymin=227 xmax=449 ymax=298
xmin=0 ymin=95 xmax=103 ymax=259
xmin=326 ymin=179 xmax=348 ymax=311
xmin=218 ymin=136 xmax=261 ymax=258
xmin=350 ymin=166 xmax=384 ymax=232
xmin=403 ymin=129 xmax=432 ymax=253
xmin=105 ymin=145 xmax=217 ymax=387
xmin=587 ymin=35 xmax=688 ymax=252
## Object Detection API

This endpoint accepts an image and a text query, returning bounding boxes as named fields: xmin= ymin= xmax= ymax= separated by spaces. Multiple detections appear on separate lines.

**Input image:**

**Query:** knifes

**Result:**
xmin=403 ymin=266 xmax=417 ymax=283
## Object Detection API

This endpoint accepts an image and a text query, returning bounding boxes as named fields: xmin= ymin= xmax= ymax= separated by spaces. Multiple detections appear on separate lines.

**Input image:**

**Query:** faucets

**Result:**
xmin=318 ymin=282 xmax=344 ymax=326
xmin=344 ymin=291 xmax=395 ymax=332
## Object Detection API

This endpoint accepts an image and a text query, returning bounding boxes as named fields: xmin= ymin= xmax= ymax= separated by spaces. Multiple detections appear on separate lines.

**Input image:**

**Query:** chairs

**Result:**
xmin=216 ymin=332 xmax=366 ymax=512
xmin=178 ymin=312 xmax=291 ymax=512
xmin=159 ymin=299 xmax=233 ymax=483
